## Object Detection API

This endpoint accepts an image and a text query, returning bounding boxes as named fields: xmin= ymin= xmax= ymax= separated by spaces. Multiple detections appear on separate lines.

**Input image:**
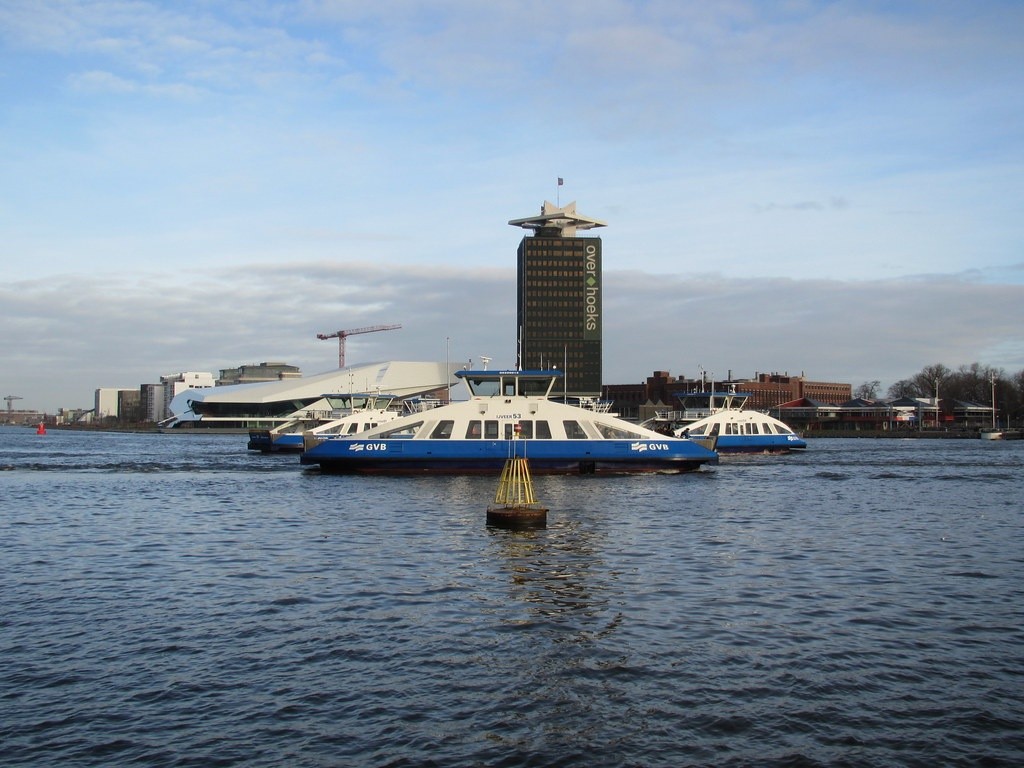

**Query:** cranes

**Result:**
xmin=317 ymin=323 xmax=401 ymax=368
xmin=3 ymin=394 xmax=23 ymax=409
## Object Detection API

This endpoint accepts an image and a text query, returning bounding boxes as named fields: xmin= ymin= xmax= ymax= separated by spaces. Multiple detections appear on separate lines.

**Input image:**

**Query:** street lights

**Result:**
xmin=779 ymin=376 xmax=786 ymax=420
xmin=934 ymin=377 xmax=940 ymax=427
xmin=988 ymin=370 xmax=998 ymax=428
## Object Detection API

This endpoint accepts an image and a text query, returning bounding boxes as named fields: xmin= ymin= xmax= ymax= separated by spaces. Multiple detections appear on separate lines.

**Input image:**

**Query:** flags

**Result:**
xmin=558 ymin=178 xmax=563 ymax=185
